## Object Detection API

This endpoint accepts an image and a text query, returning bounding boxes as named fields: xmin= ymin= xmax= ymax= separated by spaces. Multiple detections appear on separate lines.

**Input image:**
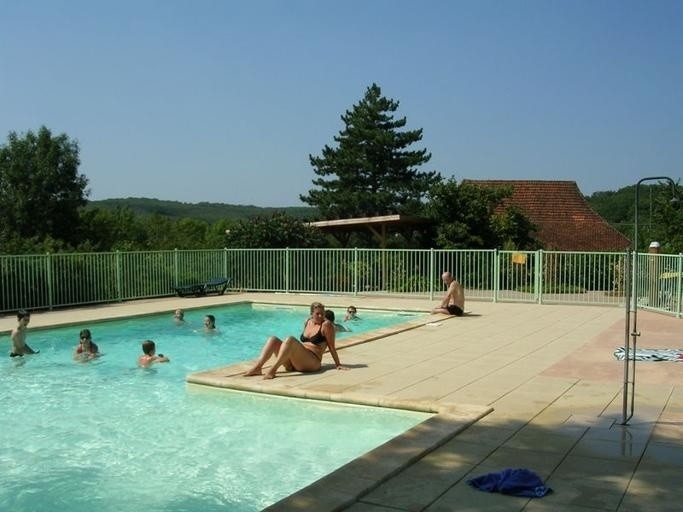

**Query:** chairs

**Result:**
xmin=169 ymin=277 xmax=234 ymax=298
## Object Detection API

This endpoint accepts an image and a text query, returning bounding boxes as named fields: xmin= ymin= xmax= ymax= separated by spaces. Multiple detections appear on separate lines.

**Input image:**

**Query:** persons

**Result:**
xmin=429 ymin=271 xmax=465 ymax=317
xmin=135 ymin=339 xmax=170 ymax=368
xmin=172 ymin=307 xmax=185 ymax=324
xmin=201 ymin=314 xmax=221 ymax=334
xmin=323 ymin=310 xmax=352 ymax=334
xmin=9 ymin=309 xmax=39 ymax=357
xmin=240 ymin=300 xmax=350 ymax=379
xmin=342 ymin=305 xmax=361 ymax=323
xmin=71 ymin=329 xmax=104 ymax=363
